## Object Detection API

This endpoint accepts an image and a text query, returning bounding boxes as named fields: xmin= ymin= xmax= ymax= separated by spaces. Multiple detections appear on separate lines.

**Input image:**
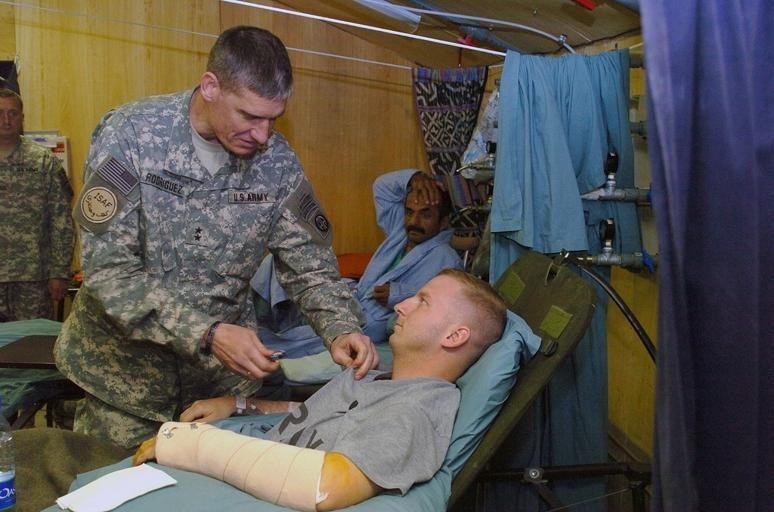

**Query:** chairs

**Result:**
xmin=447 ymin=251 xmax=597 ymax=512
xmin=0 ymin=318 xmax=394 ymax=416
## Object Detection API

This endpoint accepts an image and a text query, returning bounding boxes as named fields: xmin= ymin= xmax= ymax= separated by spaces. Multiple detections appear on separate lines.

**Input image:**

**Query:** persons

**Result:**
xmin=251 ymin=169 xmax=465 ymax=357
xmin=50 ymin=24 xmax=380 ymax=447
xmin=0 ymin=85 xmax=76 ymax=323
xmin=36 ymin=267 xmax=508 ymax=512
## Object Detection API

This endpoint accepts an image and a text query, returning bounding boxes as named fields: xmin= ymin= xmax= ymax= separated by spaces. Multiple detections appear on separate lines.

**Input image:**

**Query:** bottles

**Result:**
xmin=0 ymin=413 xmax=16 ymax=512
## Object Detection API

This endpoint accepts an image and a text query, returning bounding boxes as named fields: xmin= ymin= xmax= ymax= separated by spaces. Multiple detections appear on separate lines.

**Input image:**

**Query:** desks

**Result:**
xmin=0 ymin=336 xmax=82 ymax=430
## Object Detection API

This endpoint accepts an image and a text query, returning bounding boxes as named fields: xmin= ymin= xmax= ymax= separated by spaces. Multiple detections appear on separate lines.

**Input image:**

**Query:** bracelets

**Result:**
xmin=203 ymin=321 xmax=220 ymax=354
xmin=234 ymin=393 xmax=247 ymax=415
